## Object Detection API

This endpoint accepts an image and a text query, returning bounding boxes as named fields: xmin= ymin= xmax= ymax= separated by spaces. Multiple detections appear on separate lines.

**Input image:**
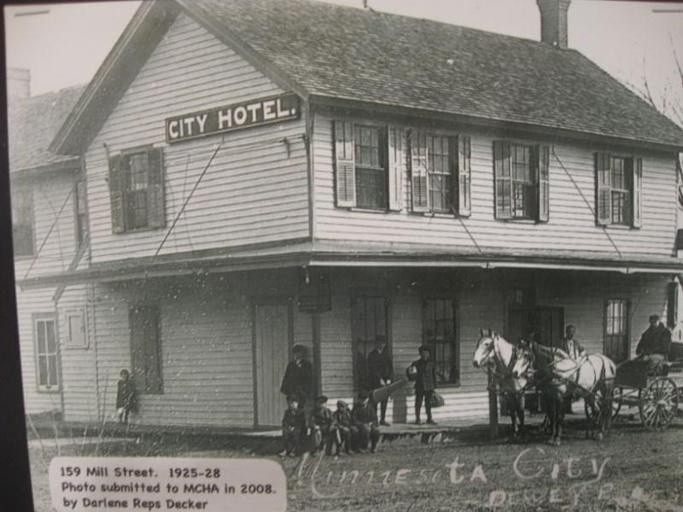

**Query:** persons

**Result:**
xmin=635 ymin=314 xmax=671 ymax=361
xmin=111 ymin=370 xmax=135 ymax=431
xmin=333 ymin=400 xmax=361 ymax=456
xmin=366 ymin=337 xmax=392 ymax=427
xmin=278 ymin=344 xmax=311 ymax=409
xmin=350 ymin=389 xmax=379 ymax=454
xmin=405 ymin=346 xmax=438 ymax=425
xmin=307 ymin=397 xmax=334 ymax=460
xmin=524 ymin=326 xmax=540 ymax=417
xmin=278 ymin=394 xmax=305 ymax=458
xmin=557 ymin=324 xmax=582 ymax=415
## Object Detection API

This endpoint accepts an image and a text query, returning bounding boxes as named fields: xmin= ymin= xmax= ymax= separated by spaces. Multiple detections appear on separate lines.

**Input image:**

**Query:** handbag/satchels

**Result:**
xmin=430 ymin=392 xmax=444 ymax=407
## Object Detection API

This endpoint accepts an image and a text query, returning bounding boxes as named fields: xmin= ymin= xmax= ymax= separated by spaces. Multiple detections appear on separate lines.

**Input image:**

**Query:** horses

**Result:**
xmin=512 ymin=330 xmax=617 ymax=446
xmin=472 ymin=326 xmax=570 ymax=436
